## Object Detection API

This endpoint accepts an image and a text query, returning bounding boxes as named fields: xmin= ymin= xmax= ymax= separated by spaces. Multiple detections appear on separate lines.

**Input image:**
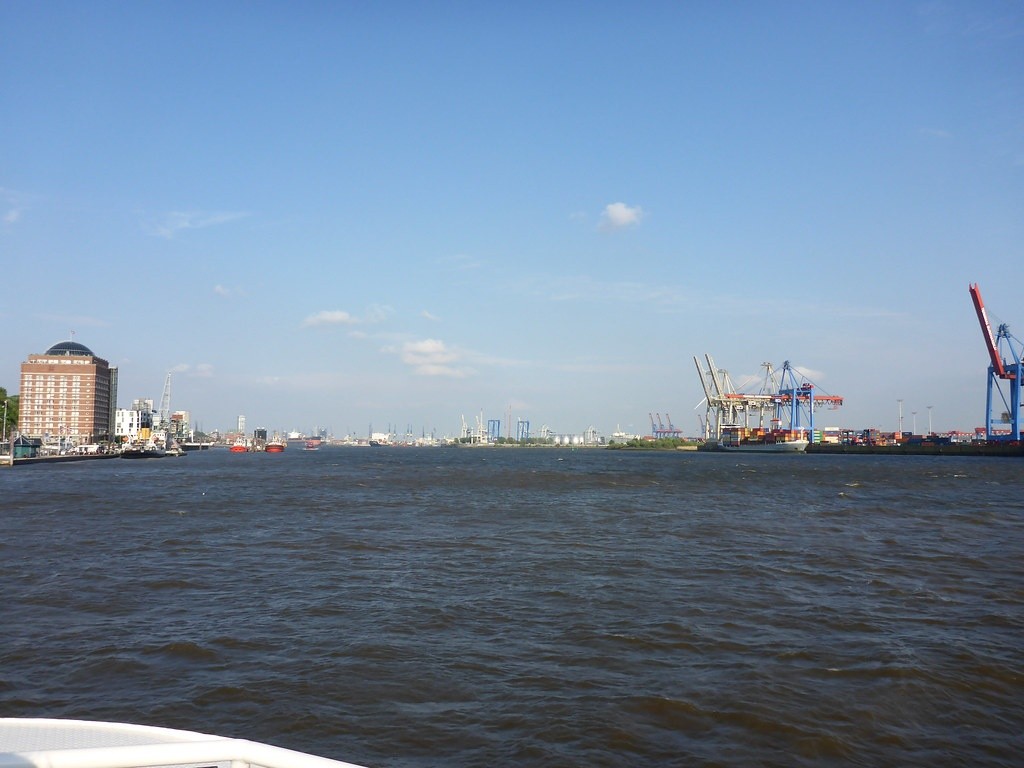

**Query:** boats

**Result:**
xmin=266 ymin=434 xmax=284 ymax=453
xmin=119 ymin=438 xmax=189 ymax=459
xmin=305 ymin=443 xmax=315 ymax=450
xmin=230 ymin=436 xmax=248 ymax=454
xmin=718 ymin=438 xmax=810 ymax=453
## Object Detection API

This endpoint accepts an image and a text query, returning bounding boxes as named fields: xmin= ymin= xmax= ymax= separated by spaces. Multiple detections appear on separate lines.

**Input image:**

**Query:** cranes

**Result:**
xmin=967 ymin=283 xmax=1024 ymax=444
xmin=691 ymin=352 xmax=841 ymax=442
xmin=649 ymin=412 xmax=682 ymax=438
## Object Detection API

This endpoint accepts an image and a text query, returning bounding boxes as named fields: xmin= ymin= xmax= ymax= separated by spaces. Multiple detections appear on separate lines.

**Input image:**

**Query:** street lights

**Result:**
xmin=895 ymin=398 xmax=904 ymax=432
xmin=911 ymin=411 xmax=918 ymax=434
xmin=926 ymin=406 xmax=934 ymax=437
